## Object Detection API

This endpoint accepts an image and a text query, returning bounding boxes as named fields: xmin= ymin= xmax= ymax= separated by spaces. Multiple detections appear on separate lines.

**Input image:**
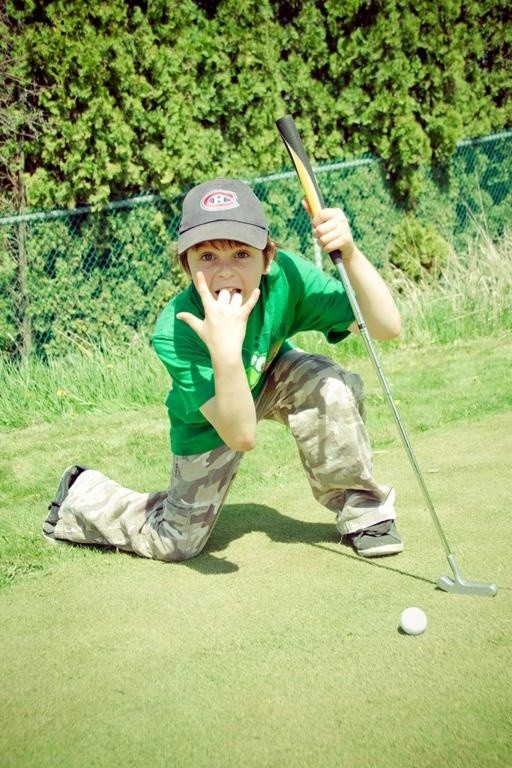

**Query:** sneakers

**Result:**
xmin=40 ymin=464 xmax=89 ymax=544
xmin=350 ymin=519 xmax=404 ymax=558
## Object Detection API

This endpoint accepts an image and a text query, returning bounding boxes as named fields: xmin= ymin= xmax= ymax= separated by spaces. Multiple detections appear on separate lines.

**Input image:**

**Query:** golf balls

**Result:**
xmin=401 ymin=607 xmax=426 ymax=634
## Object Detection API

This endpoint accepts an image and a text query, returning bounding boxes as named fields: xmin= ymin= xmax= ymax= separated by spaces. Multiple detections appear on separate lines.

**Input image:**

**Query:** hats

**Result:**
xmin=176 ymin=176 xmax=270 ymax=255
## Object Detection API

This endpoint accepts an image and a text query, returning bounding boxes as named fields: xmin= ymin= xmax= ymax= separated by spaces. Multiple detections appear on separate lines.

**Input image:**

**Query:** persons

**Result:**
xmin=41 ymin=176 xmax=406 ymax=564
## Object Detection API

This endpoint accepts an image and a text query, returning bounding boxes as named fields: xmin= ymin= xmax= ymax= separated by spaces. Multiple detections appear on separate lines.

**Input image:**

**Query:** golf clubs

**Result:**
xmin=275 ymin=116 xmax=497 ymax=598
